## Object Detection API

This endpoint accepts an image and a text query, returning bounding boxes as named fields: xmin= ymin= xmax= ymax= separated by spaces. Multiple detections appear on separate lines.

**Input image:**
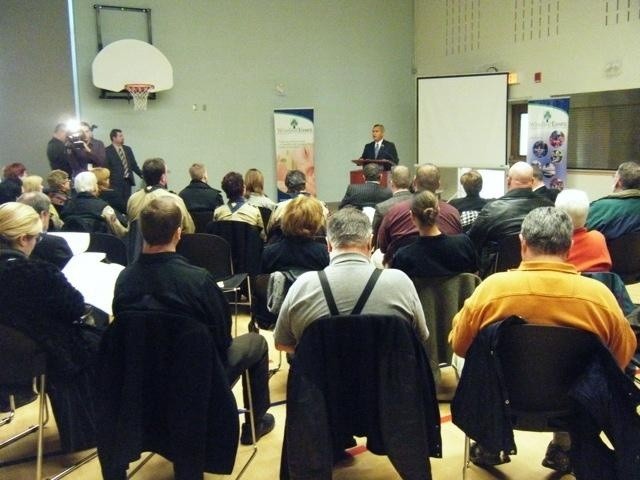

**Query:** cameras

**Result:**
xmin=66 ymin=119 xmax=86 ymax=150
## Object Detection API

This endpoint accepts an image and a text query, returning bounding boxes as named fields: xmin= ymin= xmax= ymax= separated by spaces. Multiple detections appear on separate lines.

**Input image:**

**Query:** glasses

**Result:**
xmin=65 ymin=180 xmax=71 ymax=182
xmin=48 ymin=212 xmax=54 ymax=217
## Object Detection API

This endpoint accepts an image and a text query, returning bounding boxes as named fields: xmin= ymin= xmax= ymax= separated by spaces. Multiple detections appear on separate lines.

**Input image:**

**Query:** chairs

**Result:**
xmin=491 ymin=231 xmax=522 ymax=273
xmin=288 ymin=315 xmax=433 ymax=480
xmin=461 ymin=322 xmax=601 ymax=478
xmin=88 ymin=235 xmax=129 ymax=270
xmin=208 ymin=220 xmax=262 ymax=294
xmin=1 ymin=318 xmax=97 ymax=480
xmin=254 ymin=268 xmax=312 ymax=331
xmin=411 ymin=274 xmax=482 ymax=366
xmin=91 ymin=312 xmax=258 ymax=480
xmin=178 ymin=234 xmax=255 ymax=335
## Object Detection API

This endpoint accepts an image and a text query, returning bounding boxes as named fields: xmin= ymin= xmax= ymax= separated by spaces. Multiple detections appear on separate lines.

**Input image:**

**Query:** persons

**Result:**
xmin=255 ymin=192 xmax=331 ymax=274
xmin=551 ymin=188 xmax=611 ymax=273
xmin=125 ymin=157 xmax=194 ymax=235
xmin=531 ymin=163 xmax=551 ymax=200
xmin=389 ymin=190 xmax=478 ymax=276
xmin=47 ymin=124 xmax=80 ymax=176
xmin=20 ymin=174 xmax=64 ymax=231
xmin=91 ymin=166 xmax=124 ymax=211
xmin=58 ymin=172 xmax=124 ymax=235
xmin=376 ymin=163 xmax=468 ymax=253
xmin=273 ymin=206 xmax=429 ymax=356
xmin=464 ymin=161 xmax=554 ymax=253
xmin=583 ymin=161 xmax=639 ymax=243
xmin=0 ymin=177 xmax=22 ymax=202
xmin=450 ymin=206 xmax=639 ymax=474
xmin=177 ymin=163 xmax=224 ymax=214
xmin=338 ymin=164 xmax=395 ymax=214
xmin=43 ymin=170 xmax=73 ymax=215
xmin=1 ymin=162 xmax=28 ymax=186
xmin=211 ymin=172 xmax=269 ymax=241
xmin=16 ymin=192 xmax=74 ymax=270
xmin=1 ymin=200 xmax=108 ymax=395
xmin=243 ymin=168 xmax=279 ymax=213
xmin=355 ymin=125 xmax=400 ymax=168
xmin=65 ymin=121 xmax=106 ymax=173
xmin=105 ymin=129 xmax=144 ymax=210
xmin=278 ymin=170 xmax=314 ymax=200
xmin=445 ymin=172 xmax=500 ymax=215
xmin=370 ymin=165 xmax=422 ymax=242
xmin=113 ymin=199 xmax=277 ymax=447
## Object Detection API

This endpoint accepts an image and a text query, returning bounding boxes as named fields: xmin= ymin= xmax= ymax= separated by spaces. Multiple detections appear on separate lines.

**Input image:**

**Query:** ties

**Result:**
xmin=374 ymin=144 xmax=380 ymax=160
xmin=118 ymin=148 xmax=129 ymax=178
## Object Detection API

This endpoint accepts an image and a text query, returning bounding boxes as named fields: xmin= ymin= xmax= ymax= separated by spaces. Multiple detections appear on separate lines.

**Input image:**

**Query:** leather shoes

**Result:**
xmin=241 ymin=414 xmax=275 ymax=444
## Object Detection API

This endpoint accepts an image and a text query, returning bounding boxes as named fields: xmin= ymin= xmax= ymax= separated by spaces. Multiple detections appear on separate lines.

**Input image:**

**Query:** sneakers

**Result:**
xmin=468 ymin=441 xmax=511 ymax=467
xmin=541 ymin=440 xmax=572 ymax=473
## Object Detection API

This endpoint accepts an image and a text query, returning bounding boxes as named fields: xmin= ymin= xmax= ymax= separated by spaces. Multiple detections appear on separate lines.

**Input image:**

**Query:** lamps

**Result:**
xmin=66 ymin=0 xmax=83 ymax=123
